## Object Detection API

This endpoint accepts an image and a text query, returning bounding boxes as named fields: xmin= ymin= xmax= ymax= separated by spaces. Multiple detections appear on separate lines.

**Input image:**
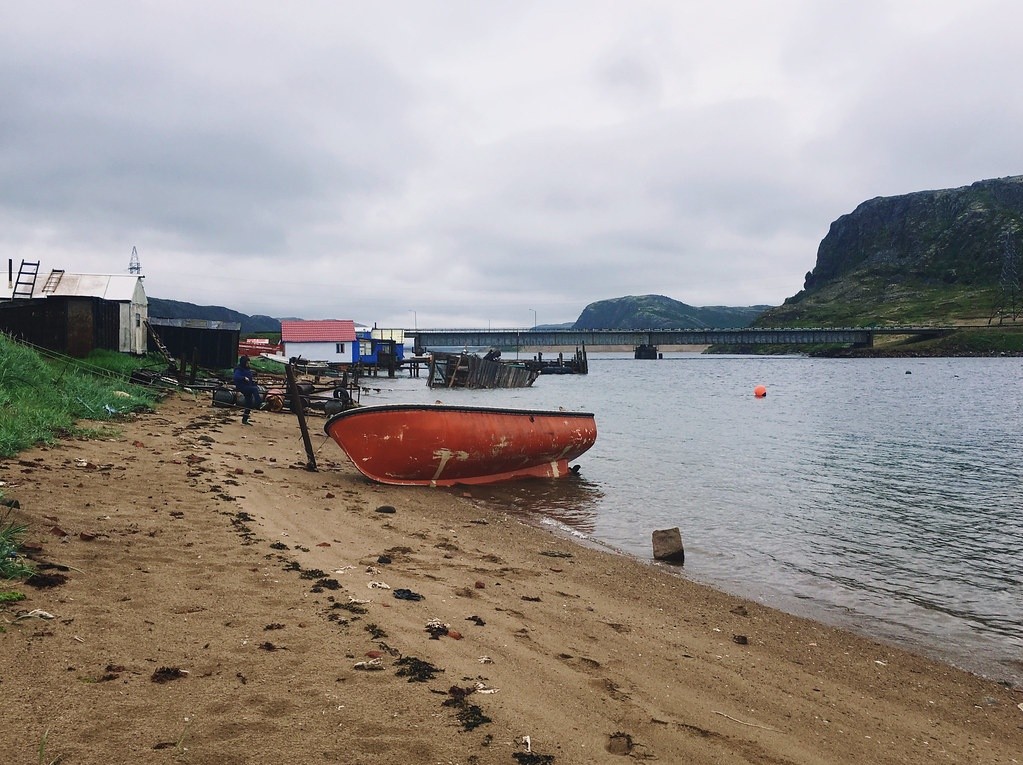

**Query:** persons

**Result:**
xmin=234 ymin=355 xmax=268 ymax=424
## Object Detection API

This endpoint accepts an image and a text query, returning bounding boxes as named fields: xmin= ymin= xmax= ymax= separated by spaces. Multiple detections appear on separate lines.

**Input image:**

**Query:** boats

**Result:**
xmin=324 ymin=403 xmax=597 ymax=486
xmin=260 ymin=352 xmax=329 ymax=375
xmin=633 ymin=344 xmax=659 ymax=359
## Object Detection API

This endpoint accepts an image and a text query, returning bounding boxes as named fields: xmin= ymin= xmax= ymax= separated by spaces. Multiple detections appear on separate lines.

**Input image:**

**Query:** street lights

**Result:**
xmin=529 ymin=309 xmax=536 ymax=331
xmin=408 ymin=310 xmax=416 ymax=329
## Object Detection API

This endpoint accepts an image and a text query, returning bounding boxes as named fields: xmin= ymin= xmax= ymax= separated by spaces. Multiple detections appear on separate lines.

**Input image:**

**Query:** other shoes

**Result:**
xmin=242 ymin=421 xmax=252 ymax=426
xmin=260 ymin=403 xmax=266 ymax=409
